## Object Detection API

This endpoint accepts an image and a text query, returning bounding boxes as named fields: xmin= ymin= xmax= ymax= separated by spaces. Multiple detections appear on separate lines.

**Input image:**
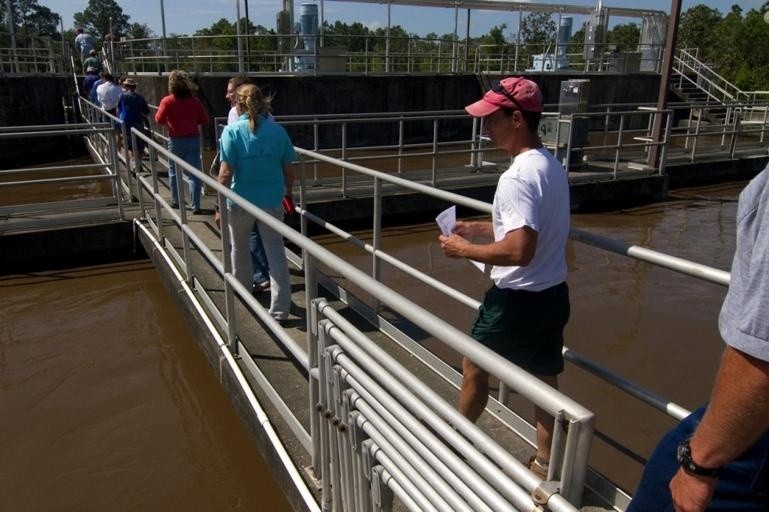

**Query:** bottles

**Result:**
xmin=285 ymin=198 xmax=296 ymax=215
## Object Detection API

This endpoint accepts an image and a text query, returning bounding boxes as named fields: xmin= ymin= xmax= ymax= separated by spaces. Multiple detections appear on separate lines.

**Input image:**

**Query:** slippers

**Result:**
xmin=494 ymin=82 xmax=529 ymax=116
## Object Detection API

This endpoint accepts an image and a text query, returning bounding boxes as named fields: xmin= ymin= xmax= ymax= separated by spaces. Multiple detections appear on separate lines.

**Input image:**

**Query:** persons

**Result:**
xmin=220 ymin=85 xmax=299 ymax=324
xmin=157 ymin=69 xmax=208 ymax=213
xmin=72 ymin=28 xmax=151 ymax=175
xmin=219 ymin=77 xmax=272 ymax=293
xmin=438 ymin=78 xmax=573 ymax=484
xmin=625 ymin=159 xmax=768 ymax=512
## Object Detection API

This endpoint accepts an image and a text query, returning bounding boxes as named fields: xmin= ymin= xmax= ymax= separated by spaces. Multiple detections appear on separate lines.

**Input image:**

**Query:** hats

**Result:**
xmin=122 ymin=78 xmax=137 ymax=86
xmin=464 ymin=76 xmax=544 ymax=116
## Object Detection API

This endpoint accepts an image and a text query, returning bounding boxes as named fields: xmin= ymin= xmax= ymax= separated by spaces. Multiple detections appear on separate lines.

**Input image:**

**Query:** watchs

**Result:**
xmin=677 ymin=440 xmax=725 ymax=477
